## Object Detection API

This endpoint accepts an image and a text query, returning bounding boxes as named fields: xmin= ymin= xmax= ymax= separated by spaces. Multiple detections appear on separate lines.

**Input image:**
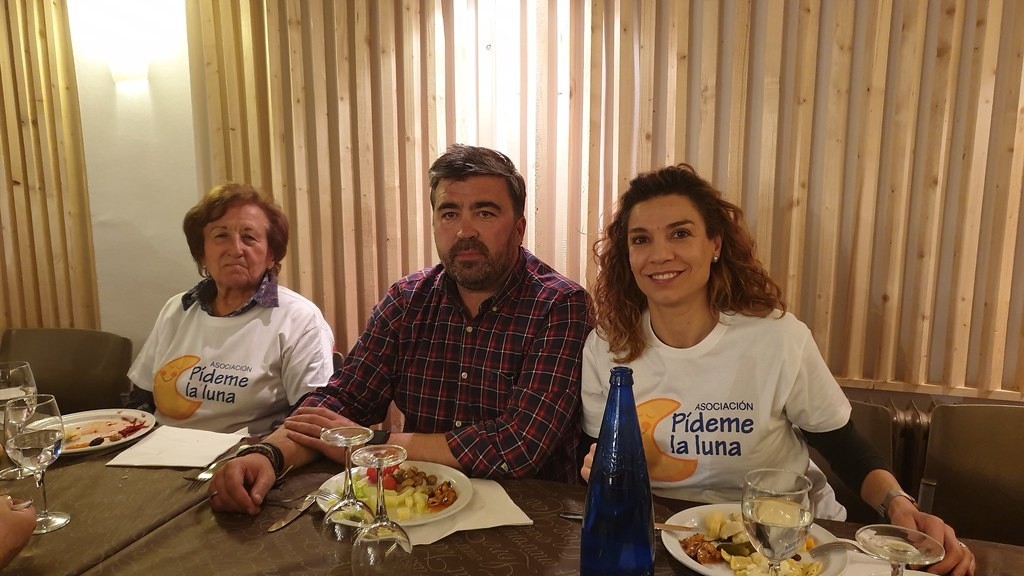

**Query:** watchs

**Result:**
xmin=365 ymin=430 xmax=391 ymax=447
xmin=879 ymin=489 xmax=921 ymax=519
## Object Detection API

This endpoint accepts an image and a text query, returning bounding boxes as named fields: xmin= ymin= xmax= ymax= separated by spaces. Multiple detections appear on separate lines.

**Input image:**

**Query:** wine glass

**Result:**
xmin=741 ymin=469 xmax=815 ymax=576
xmin=0 ymin=361 xmax=38 ymax=481
xmin=4 ymin=395 xmax=71 ymax=534
xmin=320 ymin=426 xmax=375 ymax=569
xmin=351 ymin=445 xmax=412 ymax=576
xmin=855 ymin=524 xmax=945 ymax=576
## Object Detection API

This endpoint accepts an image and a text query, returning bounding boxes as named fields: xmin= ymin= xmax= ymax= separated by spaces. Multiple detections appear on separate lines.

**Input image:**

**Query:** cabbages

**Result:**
xmin=327 ymin=467 xmax=373 ymax=518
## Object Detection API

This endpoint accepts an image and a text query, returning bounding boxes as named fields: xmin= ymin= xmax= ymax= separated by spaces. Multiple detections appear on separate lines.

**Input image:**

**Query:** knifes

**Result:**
xmin=559 ymin=511 xmax=701 ymax=532
xmin=267 ymin=491 xmax=316 ymax=531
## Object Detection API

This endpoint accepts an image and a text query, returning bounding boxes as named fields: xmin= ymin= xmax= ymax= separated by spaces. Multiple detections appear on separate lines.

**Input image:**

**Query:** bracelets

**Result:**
xmin=236 ymin=442 xmax=284 ymax=476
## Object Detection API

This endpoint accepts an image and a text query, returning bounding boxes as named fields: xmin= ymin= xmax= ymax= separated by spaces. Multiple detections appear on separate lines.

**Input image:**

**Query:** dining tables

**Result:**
xmin=0 ymin=408 xmax=1024 ymax=576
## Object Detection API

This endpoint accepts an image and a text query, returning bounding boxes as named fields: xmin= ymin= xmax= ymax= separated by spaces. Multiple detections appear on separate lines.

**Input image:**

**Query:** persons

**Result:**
xmin=208 ymin=142 xmax=598 ymax=522
xmin=124 ymin=182 xmax=336 ymax=440
xmin=581 ymin=162 xmax=976 ymax=576
xmin=0 ymin=495 xmax=37 ymax=570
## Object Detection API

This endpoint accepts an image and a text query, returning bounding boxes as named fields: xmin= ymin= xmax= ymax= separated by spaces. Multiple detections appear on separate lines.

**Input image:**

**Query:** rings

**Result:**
xmin=959 ymin=542 xmax=967 ymax=547
xmin=320 ymin=428 xmax=325 ymax=437
xmin=210 ymin=491 xmax=218 ymax=499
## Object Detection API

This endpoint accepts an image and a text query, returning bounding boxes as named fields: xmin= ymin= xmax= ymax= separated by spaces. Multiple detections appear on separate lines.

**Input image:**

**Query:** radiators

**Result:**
xmin=861 ymin=394 xmax=972 ymax=501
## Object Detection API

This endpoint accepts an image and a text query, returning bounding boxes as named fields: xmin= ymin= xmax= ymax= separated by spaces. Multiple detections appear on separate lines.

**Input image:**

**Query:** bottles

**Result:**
xmin=580 ymin=366 xmax=653 ymax=576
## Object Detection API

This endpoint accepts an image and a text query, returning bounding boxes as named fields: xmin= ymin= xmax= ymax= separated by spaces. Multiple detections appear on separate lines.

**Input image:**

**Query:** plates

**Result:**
xmin=25 ymin=409 xmax=155 ymax=456
xmin=317 ymin=460 xmax=473 ymax=526
xmin=662 ymin=503 xmax=849 ymax=576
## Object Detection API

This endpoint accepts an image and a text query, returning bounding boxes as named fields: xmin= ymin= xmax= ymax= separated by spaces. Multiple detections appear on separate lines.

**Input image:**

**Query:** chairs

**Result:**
xmin=917 ymin=405 xmax=1024 ymax=547
xmin=809 ymin=400 xmax=893 ymax=524
xmin=0 ymin=328 xmax=133 ymax=415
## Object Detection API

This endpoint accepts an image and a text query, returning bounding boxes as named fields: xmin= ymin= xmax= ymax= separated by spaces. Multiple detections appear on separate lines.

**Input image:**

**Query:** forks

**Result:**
xmin=182 ymin=445 xmax=251 ymax=481
xmin=262 ymin=490 xmax=341 ymax=503
xmin=809 ymin=542 xmax=881 ymax=559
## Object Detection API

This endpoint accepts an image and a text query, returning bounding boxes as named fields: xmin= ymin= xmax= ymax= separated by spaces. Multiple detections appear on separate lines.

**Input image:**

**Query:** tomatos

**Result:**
xmin=370 ymin=465 xmax=401 ymax=492
